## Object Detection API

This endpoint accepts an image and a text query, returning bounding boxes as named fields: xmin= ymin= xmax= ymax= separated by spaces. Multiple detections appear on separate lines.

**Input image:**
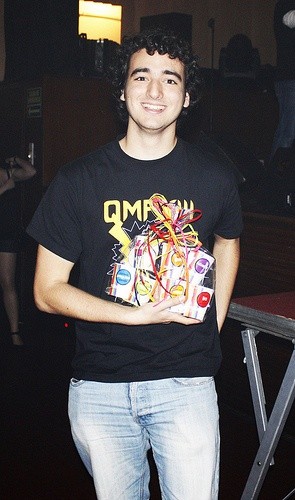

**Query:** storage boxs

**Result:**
xmin=108 ymin=262 xmax=214 ymax=322
xmin=134 ymin=234 xmax=215 ymax=285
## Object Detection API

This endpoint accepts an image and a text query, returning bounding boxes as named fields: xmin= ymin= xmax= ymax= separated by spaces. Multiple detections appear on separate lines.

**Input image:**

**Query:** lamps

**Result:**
xmin=78 ymin=0 xmax=123 ymax=79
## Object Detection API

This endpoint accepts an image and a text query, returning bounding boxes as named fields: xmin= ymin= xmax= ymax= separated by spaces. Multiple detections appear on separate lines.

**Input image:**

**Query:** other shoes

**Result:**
xmin=10 ymin=332 xmax=25 ymax=354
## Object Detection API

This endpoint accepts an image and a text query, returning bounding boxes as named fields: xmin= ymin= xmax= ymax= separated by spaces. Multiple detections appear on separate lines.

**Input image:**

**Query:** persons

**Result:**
xmin=215 ymin=0 xmax=295 ymax=164
xmin=24 ymin=27 xmax=245 ymax=500
xmin=0 ymin=142 xmax=38 ymax=351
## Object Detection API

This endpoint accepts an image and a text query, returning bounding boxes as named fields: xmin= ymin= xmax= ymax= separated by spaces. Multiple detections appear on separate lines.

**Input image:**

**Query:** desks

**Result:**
xmin=227 ymin=291 xmax=295 ymax=500
